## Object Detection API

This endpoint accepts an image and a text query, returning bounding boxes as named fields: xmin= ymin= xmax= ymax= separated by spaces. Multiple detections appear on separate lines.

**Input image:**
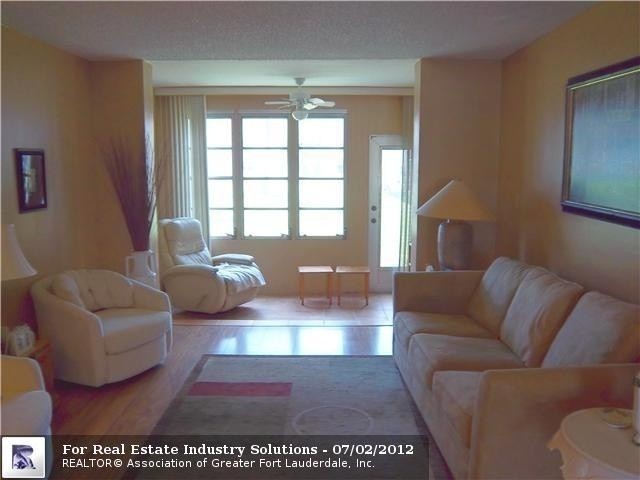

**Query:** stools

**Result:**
xmin=297 ymin=264 xmax=371 ymax=307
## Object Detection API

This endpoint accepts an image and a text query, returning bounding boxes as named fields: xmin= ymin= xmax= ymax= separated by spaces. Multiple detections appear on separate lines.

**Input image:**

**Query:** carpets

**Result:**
xmin=116 ymin=353 xmax=453 ymax=479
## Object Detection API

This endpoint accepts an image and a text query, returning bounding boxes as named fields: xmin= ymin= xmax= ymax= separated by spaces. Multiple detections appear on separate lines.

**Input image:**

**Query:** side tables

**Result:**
xmin=560 ymin=408 xmax=639 ymax=480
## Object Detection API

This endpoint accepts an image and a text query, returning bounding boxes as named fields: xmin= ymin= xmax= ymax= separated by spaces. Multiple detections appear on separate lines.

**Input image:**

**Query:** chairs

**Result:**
xmin=2 ymin=355 xmax=53 ymax=478
xmin=30 ymin=267 xmax=170 ymax=388
xmin=158 ymin=217 xmax=263 ymax=315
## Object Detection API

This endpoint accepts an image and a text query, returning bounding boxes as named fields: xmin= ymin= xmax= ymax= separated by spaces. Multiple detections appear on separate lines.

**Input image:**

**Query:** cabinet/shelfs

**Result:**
xmin=16 ymin=338 xmax=58 ymax=408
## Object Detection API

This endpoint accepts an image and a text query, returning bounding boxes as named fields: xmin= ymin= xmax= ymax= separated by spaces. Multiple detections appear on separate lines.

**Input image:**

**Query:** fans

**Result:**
xmin=262 ymin=78 xmax=337 ymax=111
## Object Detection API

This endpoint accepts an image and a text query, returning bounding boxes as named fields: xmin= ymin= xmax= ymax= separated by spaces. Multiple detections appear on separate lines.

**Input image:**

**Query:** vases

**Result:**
xmin=125 ymin=250 xmax=158 ymax=289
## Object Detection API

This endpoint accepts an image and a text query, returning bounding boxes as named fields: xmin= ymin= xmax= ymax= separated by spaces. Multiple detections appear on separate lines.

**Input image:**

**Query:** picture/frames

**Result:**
xmin=15 ymin=148 xmax=48 ymax=213
xmin=561 ymin=63 xmax=639 ymax=228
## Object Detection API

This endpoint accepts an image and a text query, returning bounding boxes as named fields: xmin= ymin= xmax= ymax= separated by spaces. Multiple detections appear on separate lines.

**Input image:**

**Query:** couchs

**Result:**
xmin=392 ymin=255 xmax=639 ymax=480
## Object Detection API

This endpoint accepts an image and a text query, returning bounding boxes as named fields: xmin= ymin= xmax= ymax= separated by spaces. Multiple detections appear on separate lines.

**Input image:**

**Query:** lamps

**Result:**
xmin=291 ymin=99 xmax=308 ymax=121
xmin=416 ymin=180 xmax=495 ymax=270
xmin=2 ymin=226 xmax=35 ymax=290
